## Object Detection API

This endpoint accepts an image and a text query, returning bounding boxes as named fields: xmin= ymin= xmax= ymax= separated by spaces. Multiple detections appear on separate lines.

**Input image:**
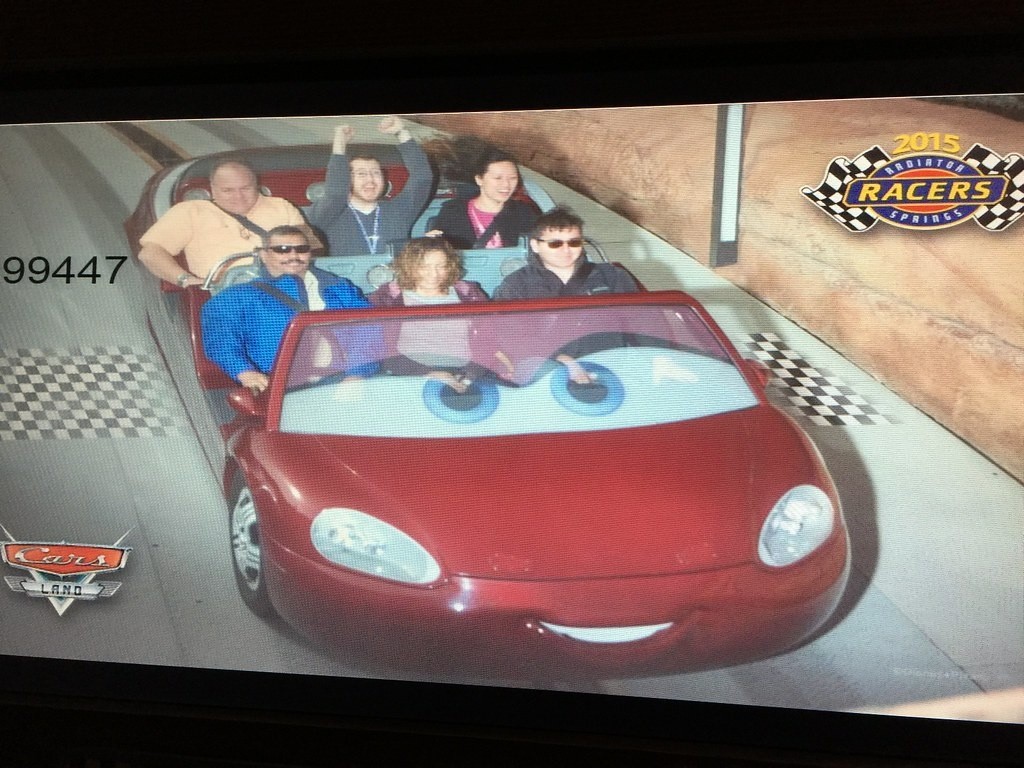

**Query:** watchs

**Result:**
xmin=178 ymin=273 xmax=192 ymax=288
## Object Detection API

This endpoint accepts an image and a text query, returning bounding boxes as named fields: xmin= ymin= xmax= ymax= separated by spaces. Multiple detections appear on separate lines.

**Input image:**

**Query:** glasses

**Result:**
xmin=265 ymin=245 xmax=311 ymax=254
xmin=533 ymin=238 xmax=583 ymax=248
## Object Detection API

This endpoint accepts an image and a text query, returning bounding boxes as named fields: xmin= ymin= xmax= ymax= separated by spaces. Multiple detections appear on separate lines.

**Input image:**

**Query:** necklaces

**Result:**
xmin=350 ymin=201 xmax=380 ymax=253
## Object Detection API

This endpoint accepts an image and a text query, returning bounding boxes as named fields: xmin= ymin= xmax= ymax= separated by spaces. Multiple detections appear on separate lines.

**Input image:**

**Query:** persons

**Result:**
xmin=139 ymin=163 xmax=304 ymax=288
xmin=496 ymin=208 xmax=669 ymax=384
xmin=201 ymin=227 xmax=386 ymax=395
xmin=370 ymin=241 xmax=499 ymax=391
xmin=425 ymin=137 xmax=541 ymax=248
xmin=312 ymin=116 xmax=435 ymax=255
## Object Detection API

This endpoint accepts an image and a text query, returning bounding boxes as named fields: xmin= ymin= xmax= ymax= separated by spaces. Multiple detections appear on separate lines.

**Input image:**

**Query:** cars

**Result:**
xmin=120 ymin=139 xmax=851 ymax=679
